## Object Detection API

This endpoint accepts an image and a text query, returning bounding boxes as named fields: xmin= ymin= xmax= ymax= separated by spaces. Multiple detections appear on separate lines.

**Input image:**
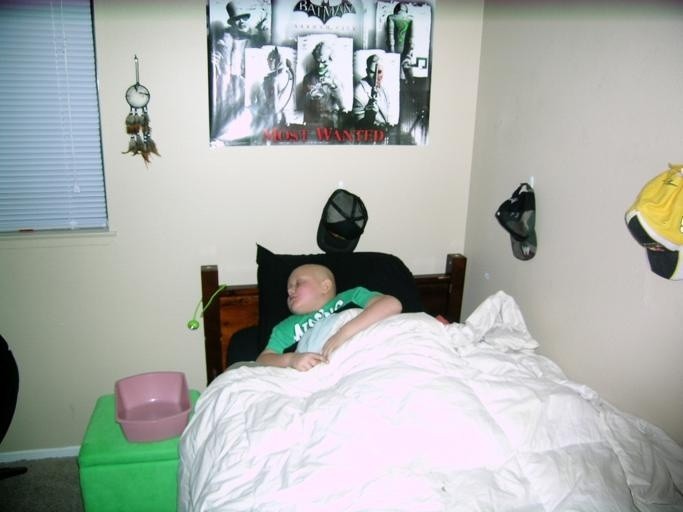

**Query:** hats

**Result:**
xmin=493 ymin=179 xmax=539 ymax=263
xmin=315 ymin=186 xmax=370 ymax=258
xmin=226 ymin=0 xmax=252 ymax=24
xmin=625 ymin=162 xmax=683 ymax=284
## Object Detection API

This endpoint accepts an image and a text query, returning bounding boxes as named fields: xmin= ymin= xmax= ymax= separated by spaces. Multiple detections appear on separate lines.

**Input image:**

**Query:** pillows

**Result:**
xmin=253 ymin=242 xmax=438 ymax=342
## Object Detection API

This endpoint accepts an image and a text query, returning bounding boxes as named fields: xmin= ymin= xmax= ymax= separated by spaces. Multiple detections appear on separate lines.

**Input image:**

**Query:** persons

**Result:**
xmin=210 ymin=1 xmax=424 ymax=146
xmin=254 ymin=263 xmax=403 ymax=373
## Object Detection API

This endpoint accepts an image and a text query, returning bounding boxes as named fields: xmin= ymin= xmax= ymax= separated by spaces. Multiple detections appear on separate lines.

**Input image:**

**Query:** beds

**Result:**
xmin=178 ymin=252 xmax=683 ymax=510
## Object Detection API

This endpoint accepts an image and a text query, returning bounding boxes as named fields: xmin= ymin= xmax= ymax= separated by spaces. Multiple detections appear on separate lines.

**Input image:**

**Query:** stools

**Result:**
xmin=77 ymin=387 xmax=204 ymax=511
